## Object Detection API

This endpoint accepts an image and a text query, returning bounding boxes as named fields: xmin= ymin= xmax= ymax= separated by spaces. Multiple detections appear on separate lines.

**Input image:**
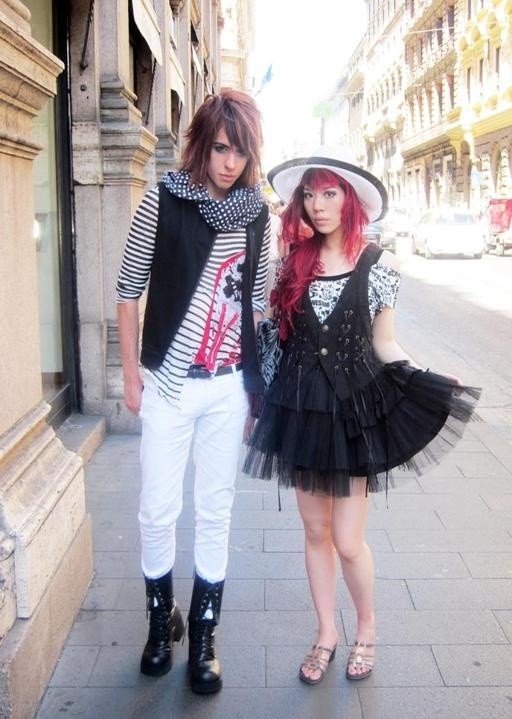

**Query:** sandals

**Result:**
xmin=299 ymin=641 xmax=337 ymax=684
xmin=346 ymin=640 xmax=376 ymax=678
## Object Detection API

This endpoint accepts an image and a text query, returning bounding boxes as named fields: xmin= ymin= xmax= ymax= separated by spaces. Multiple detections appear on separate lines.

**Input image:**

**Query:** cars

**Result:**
xmin=364 ymin=224 xmax=395 ymax=256
xmin=410 ymin=207 xmax=485 ymax=257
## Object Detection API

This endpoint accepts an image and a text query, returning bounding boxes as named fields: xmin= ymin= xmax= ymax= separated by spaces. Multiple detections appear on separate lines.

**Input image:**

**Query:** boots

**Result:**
xmin=140 ymin=567 xmax=186 ymax=676
xmin=188 ymin=572 xmax=225 ymax=693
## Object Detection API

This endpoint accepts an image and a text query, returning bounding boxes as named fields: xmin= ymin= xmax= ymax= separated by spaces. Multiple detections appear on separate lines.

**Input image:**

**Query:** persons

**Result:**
xmin=262 ymin=192 xmax=288 ymax=258
xmin=115 ymin=89 xmax=271 ymax=695
xmin=267 ymin=137 xmax=466 ymax=684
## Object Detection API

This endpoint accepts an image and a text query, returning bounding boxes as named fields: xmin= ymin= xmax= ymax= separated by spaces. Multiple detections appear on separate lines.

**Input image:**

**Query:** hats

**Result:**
xmin=267 ymin=156 xmax=388 ymax=222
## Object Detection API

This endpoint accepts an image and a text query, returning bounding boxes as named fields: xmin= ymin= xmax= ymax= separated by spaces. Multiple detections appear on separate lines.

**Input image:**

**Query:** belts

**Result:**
xmin=186 ymin=362 xmax=243 ymax=378
